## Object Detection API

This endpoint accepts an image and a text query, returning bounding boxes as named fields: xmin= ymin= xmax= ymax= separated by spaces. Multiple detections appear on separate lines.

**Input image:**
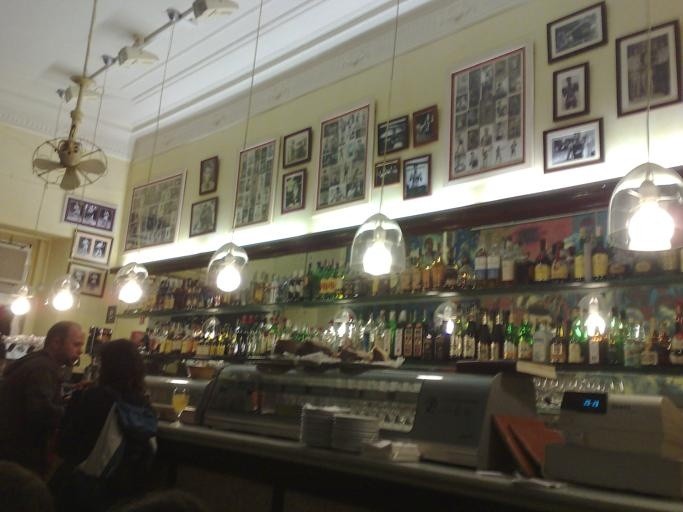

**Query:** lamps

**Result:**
xmin=44 ymin=54 xmax=108 ymax=313
xmin=608 ymin=1 xmax=682 ymax=252
xmin=202 ymin=0 xmax=272 ymax=295
xmin=112 ymin=6 xmax=183 ymax=304
xmin=348 ymin=2 xmax=410 ymax=280
xmin=4 ymin=88 xmax=68 ymax=319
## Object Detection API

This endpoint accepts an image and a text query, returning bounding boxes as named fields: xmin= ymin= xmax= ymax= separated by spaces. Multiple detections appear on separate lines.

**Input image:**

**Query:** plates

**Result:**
xmin=299 ymin=405 xmax=380 ymax=454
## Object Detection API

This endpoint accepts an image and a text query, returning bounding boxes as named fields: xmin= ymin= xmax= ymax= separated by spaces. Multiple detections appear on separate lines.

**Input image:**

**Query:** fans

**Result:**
xmin=30 ymin=74 xmax=107 ymax=192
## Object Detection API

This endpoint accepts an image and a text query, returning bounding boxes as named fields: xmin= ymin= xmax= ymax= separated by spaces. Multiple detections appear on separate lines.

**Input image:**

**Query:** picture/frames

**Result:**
xmin=199 ymin=156 xmax=219 ymax=195
xmin=232 ymin=102 xmax=439 ymax=232
xmin=614 ymin=20 xmax=682 ymax=119
xmin=546 ymin=2 xmax=609 ymax=66
xmin=189 ymin=197 xmax=219 ymax=238
xmin=443 ymin=41 xmax=528 ymax=186
xmin=543 ymin=117 xmax=605 ymax=174
xmin=553 ymin=61 xmax=589 ymax=122
xmin=56 ymin=191 xmax=119 ymax=299
xmin=124 ymin=167 xmax=187 ymax=252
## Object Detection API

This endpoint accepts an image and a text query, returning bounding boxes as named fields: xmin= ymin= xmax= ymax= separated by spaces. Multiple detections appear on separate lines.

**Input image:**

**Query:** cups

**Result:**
xmin=268 ymin=376 xmax=421 ymax=393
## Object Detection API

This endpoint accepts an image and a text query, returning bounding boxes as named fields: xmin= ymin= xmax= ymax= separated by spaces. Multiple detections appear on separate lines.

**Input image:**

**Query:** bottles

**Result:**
xmin=85 ymin=326 xmax=112 ymax=356
xmin=6 ymin=341 xmax=36 ymax=354
xmin=136 ymin=225 xmax=682 ymax=367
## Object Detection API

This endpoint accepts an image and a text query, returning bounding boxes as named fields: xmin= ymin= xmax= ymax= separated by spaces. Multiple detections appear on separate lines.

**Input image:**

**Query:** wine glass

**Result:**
xmin=532 ymin=376 xmax=625 ymax=409
xmin=170 ymin=387 xmax=191 ymax=428
xmin=278 ymin=394 xmax=416 ymax=429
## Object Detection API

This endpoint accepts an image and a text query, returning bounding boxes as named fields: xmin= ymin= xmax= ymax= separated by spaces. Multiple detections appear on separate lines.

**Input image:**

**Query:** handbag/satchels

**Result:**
xmin=112 ymin=399 xmax=160 ymax=441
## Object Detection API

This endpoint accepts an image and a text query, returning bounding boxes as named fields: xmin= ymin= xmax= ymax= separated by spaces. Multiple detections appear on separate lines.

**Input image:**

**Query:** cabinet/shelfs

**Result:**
xmin=116 ymin=265 xmax=680 ymax=379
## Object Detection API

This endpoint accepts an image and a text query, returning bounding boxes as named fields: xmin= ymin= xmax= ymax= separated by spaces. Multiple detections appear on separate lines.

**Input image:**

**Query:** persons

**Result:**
xmin=51 ymin=340 xmax=157 ymax=512
xmin=409 ymin=162 xmax=422 ymax=190
xmin=74 ymin=272 xmax=99 ymax=287
xmin=419 ymin=112 xmax=434 ymax=141
xmin=565 ymin=133 xmax=588 ymax=161
xmin=71 ymin=201 xmax=110 ymax=226
xmin=453 ymin=54 xmax=522 ymax=172
xmin=202 ymin=163 xmax=215 ymax=192
xmin=420 ymin=236 xmax=436 ymax=263
xmin=562 ymin=76 xmax=580 ymax=109
xmin=81 ymin=237 xmax=103 ymax=256
xmin=629 ymin=40 xmax=670 ymax=101
xmin=1 ymin=323 xmax=83 ymax=472
xmin=320 ymin=110 xmax=365 ymax=207
xmin=286 ymin=183 xmax=300 ymax=208
